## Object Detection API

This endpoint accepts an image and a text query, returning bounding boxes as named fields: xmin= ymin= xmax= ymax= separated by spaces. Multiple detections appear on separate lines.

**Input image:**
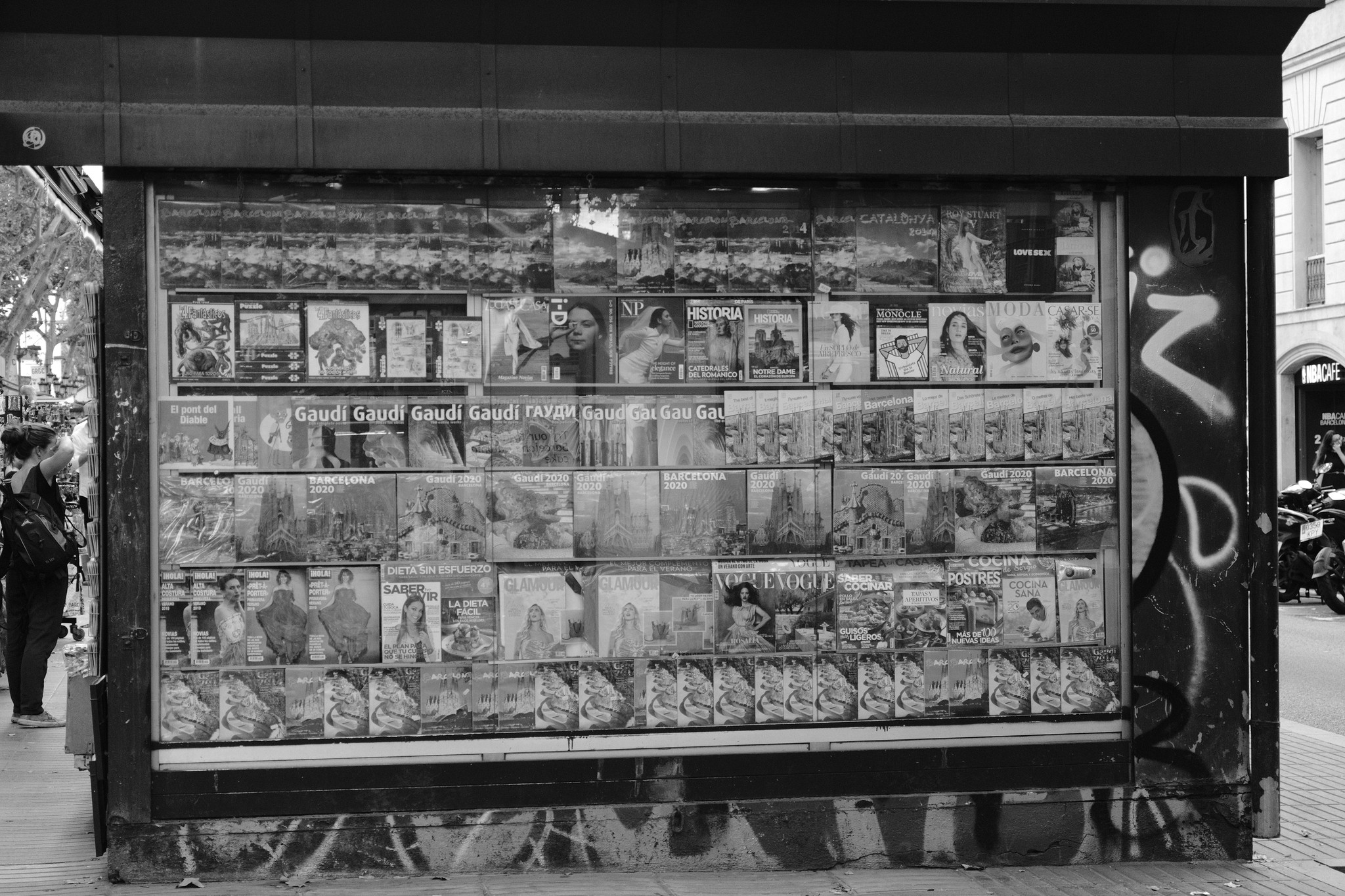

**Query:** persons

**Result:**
xmin=151 ymin=181 xmax=1121 ymax=739
xmin=68 ymin=414 xmax=95 ymax=548
xmin=605 ymin=601 xmax=648 ymax=658
xmin=718 ymin=579 xmax=776 ymax=654
xmin=999 ymin=317 xmax=1034 ymax=364
xmin=1312 ymin=430 xmax=1345 ymax=495
xmin=929 ymin=311 xmax=988 ymax=381
xmin=957 ymin=207 xmax=995 ymax=291
xmin=382 ymin=594 xmax=436 ymax=663
xmin=513 ymin=603 xmax=558 ymax=658
xmin=617 ymin=306 xmax=684 ymax=384
xmin=213 ymin=573 xmax=246 ymax=667
xmin=318 ymin=567 xmax=371 ymax=664
xmin=502 ymin=304 xmax=542 ymax=376
xmin=820 ymin=313 xmax=858 ymax=382
xmin=0 ymin=422 xmax=75 ymax=729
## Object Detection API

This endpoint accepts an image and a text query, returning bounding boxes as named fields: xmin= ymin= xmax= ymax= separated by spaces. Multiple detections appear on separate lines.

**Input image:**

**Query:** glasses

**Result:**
xmin=1332 ymin=436 xmax=1343 ymax=443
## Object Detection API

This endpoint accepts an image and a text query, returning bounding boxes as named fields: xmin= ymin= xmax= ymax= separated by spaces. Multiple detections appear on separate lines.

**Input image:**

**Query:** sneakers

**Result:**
xmin=10 ymin=710 xmax=66 ymax=727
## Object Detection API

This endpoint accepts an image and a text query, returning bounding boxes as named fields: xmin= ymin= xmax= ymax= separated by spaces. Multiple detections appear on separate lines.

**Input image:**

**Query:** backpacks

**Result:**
xmin=0 ymin=491 xmax=87 ymax=591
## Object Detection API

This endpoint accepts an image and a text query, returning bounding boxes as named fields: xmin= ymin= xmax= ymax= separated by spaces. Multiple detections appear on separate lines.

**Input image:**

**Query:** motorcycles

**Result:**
xmin=1277 ymin=462 xmax=1345 ymax=616
